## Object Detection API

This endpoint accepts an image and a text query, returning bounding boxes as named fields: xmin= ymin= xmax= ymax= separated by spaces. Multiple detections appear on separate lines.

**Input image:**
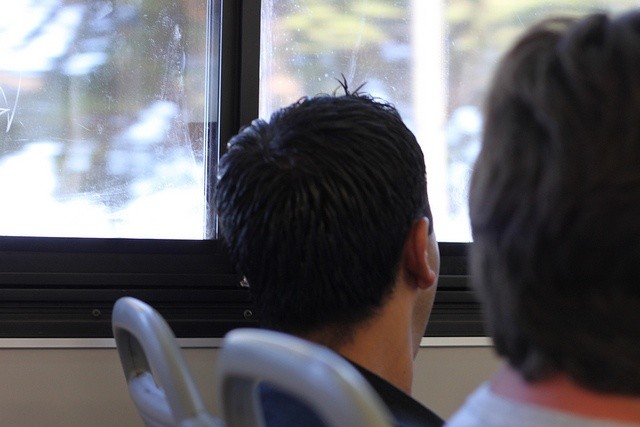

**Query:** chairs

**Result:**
xmin=112 ymin=296 xmax=222 ymax=426
xmin=218 ymin=327 xmax=404 ymax=427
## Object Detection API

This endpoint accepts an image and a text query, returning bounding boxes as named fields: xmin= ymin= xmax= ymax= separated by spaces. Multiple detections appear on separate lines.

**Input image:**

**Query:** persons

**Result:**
xmin=442 ymin=6 xmax=639 ymax=425
xmin=211 ymin=70 xmax=452 ymax=426
